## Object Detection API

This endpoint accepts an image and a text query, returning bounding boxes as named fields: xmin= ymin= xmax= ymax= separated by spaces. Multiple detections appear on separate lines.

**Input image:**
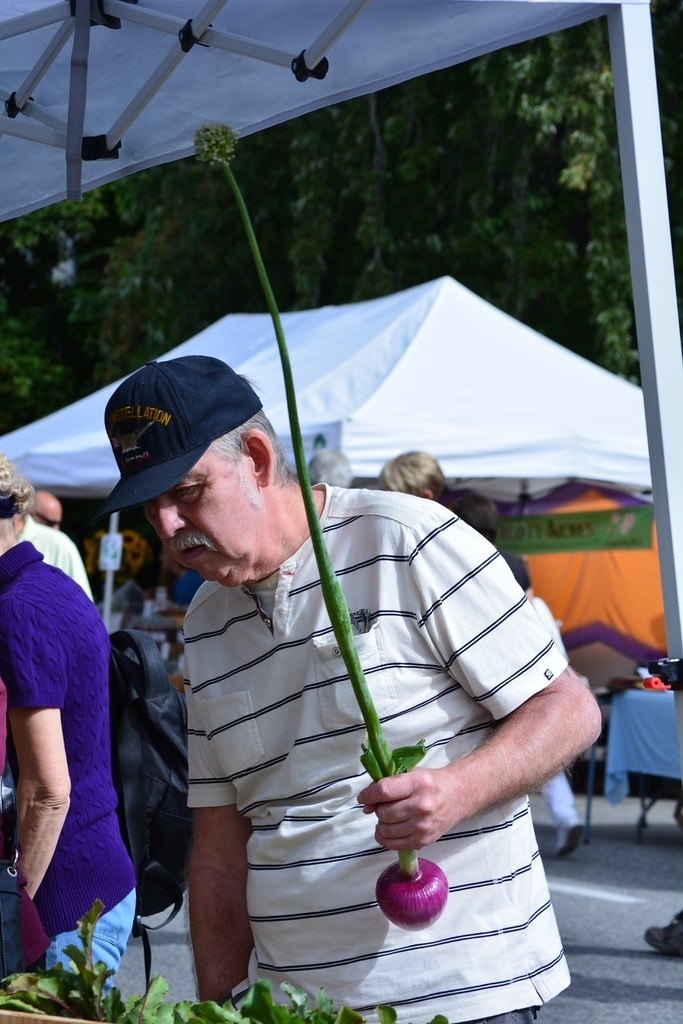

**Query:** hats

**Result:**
xmin=92 ymin=355 xmax=263 ymax=522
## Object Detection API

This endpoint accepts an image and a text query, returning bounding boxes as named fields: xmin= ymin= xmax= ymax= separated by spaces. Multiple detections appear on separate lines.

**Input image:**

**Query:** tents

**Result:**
xmin=0 ymin=275 xmax=653 ymax=633
xmin=0 ymin=0 xmax=683 ymax=732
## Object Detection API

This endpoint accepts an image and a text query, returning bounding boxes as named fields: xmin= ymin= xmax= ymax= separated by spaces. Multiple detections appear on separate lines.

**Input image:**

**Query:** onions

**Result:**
xmin=376 ymin=856 xmax=449 ymax=931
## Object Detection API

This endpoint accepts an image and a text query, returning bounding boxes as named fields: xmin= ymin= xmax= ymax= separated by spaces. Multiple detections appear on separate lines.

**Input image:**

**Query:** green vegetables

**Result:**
xmin=0 ymin=898 xmax=450 ymax=1024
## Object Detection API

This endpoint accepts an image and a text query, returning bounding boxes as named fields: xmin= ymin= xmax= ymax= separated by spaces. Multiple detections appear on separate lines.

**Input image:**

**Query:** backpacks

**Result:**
xmin=106 ymin=629 xmax=199 ymax=939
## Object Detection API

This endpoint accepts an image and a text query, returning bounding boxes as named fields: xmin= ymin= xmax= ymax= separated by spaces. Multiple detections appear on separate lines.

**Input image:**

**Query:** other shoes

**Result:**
xmin=552 ymin=817 xmax=583 ymax=858
xmin=644 ymin=919 xmax=683 ymax=958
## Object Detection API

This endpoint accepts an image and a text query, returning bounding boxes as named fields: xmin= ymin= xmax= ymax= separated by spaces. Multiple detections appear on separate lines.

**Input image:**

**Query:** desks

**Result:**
xmin=607 ymin=687 xmax=682 ymax=843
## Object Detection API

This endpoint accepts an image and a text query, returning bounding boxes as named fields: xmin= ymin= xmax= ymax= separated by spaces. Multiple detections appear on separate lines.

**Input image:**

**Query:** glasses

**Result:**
xmin=35 ymin=512 xmax=62 ymax=529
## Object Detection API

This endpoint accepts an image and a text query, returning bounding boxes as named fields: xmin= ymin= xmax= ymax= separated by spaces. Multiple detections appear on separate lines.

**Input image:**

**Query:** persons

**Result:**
xmin=30 ymin=491 xmax=62 ymax=530
xmin=105 ymin=357 xmax=600 ymax=1024
xmin=307 ymin=452 xmax=354 ymax=487
xmin=0 ymin=454 xmax=135 ymax=1001
xmin=375 ymin=452 xmax=451 ymax=508
xmin=452 ymin=486 xmax=583 ymax=857
